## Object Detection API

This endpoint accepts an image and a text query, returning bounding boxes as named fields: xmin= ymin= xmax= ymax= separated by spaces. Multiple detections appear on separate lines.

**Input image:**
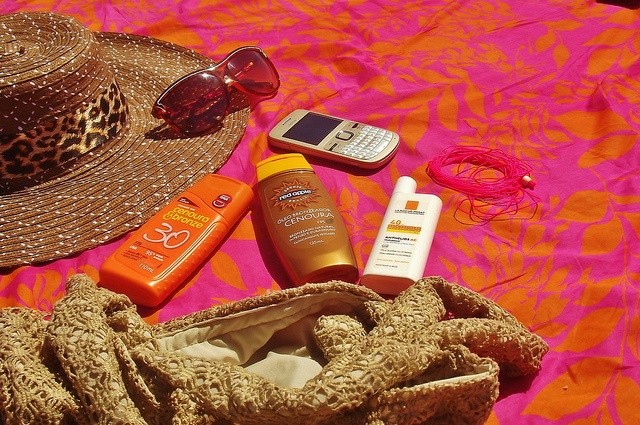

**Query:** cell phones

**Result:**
xmin=268 ymin=108 xmax=400 ymax=170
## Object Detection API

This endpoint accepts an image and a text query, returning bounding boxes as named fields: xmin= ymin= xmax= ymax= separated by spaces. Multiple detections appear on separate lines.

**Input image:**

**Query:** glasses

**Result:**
xmin=152 ymin=45 xmax=280 ymax=135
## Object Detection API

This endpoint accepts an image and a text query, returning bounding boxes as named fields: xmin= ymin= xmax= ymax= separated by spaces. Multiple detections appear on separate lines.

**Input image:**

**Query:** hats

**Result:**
xmin=0 ymin=11 xmax=251 ymax=268
